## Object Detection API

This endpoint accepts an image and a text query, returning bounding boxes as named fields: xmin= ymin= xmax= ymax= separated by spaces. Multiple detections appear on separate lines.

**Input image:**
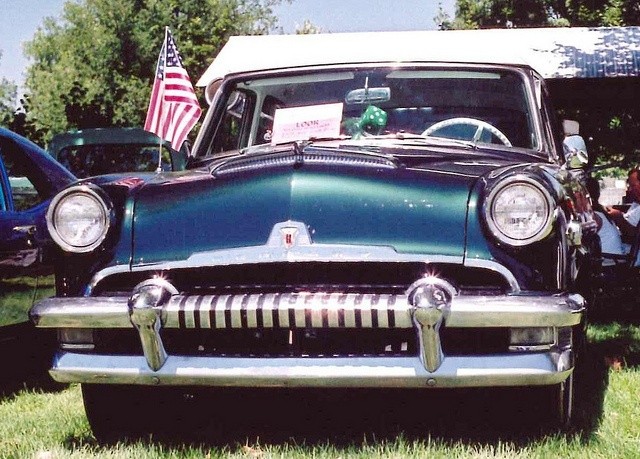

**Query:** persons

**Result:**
xmin=577 ymin=168 xmax=640 ymax=267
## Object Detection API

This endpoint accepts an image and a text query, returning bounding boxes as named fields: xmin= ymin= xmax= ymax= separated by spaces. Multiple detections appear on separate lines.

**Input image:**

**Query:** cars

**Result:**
xmin=28 ymin=61 xmax=605 ymax=445
xmin=45 ymin=127 xmax=191 ymax=175
xmin=0 ymin=125 xmax=80 ymax=329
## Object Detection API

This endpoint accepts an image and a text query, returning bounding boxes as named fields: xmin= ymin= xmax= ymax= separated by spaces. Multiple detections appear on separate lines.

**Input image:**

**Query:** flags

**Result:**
xmin=143 ymin=26 xmax=202 ymax=153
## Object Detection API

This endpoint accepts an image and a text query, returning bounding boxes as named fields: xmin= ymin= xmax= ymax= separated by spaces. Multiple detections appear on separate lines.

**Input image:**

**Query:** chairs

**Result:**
xmin=581 ymin=209 xmax=640 ymax=344
